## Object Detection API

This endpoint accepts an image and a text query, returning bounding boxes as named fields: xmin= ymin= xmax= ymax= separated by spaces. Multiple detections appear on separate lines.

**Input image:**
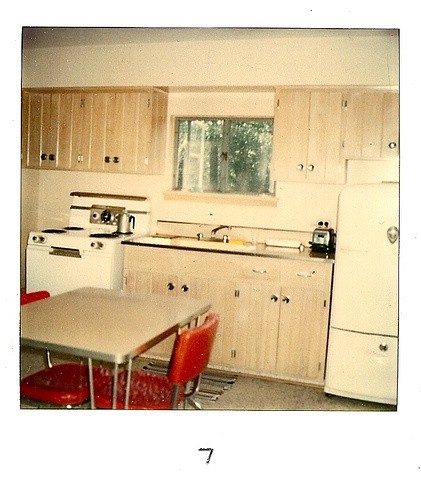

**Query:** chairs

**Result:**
xmin=21 ymin=291 xmax=113 ymax=410
xmin=96 ymin=313 xmax=219 ymax=410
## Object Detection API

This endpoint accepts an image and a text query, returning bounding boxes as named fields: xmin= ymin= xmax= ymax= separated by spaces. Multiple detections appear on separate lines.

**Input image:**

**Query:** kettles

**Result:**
xmin=115 ymin=213 xmax=136 ymax=234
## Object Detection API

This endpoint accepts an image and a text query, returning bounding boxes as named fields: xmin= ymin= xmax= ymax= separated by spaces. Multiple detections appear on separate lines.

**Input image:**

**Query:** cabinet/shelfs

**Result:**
xmin=233 ymin=253 xmax=335 ymax=387
xmin=345 ymin=85 xmax=399 ymax=162
xmin=81 ymin=86 xmax=168 ymax=176
xmin=21 ymin=86 xmax=83 ymax=171
xmin=271 ymin=85 xmax=348 ymax=185
xmin=123 ymin=244 xmax=236 ymax=386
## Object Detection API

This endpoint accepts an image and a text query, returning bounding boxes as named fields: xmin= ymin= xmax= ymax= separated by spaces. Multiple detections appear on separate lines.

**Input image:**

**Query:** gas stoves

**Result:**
xmin=27 ymin=204 xmax=131 ymax=251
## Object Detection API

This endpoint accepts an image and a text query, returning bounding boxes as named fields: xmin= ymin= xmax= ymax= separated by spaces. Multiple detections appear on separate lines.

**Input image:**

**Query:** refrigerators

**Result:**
xmin=322 ymin=180 xmax=399 ymax=409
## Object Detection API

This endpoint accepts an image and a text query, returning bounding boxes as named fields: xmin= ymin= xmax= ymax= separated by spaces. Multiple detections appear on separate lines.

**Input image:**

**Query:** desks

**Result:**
xmin=21 ymin=286 xmax=212 ymax=410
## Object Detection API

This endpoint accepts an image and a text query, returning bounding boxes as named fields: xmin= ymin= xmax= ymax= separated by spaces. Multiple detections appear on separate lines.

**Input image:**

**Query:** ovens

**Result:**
xmin=25 ymin=245 xmax=123 ymax=297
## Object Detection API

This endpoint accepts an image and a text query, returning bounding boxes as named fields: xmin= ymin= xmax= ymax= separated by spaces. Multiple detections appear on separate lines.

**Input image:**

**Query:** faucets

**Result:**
xmin=212 ymin=226 xmax=230 ymax=239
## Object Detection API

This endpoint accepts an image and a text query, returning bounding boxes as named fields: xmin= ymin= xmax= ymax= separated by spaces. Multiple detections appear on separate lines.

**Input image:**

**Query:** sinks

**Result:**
xmin=172 ymin=237 xmax=248 ymax=252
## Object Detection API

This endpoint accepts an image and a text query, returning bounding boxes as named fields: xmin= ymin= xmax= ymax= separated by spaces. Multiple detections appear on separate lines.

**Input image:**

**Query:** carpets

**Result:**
xmin=141 ymin=359 xmax=238 ymax=410
